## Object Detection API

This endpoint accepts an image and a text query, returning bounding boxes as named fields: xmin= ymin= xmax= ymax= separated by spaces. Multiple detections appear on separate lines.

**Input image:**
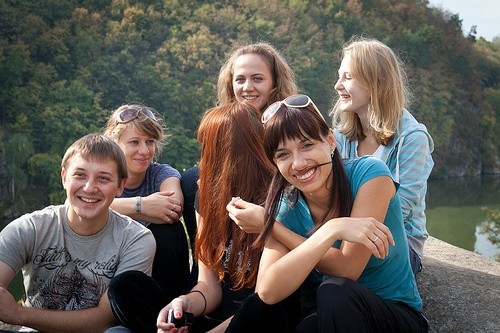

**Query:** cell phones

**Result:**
xmin=168 ymin=311 xmax=194 ymax=331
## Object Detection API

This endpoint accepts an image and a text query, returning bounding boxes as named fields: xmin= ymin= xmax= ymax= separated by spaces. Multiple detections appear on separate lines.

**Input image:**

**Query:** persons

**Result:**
xmin=105 ymin=43 xmax=299 ymax=293
xmin=330 ymin=39 xmax=436 ymax=275
xmin=222 ymin=98 xmax=430 ymax=333
xmin=106 ymin=101 xmax=277 ymax=333
xmin=0 ymin=134 xmax=157 ymax=333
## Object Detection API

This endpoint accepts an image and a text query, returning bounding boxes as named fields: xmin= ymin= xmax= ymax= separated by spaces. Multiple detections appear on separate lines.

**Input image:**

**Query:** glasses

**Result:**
xmin=113 ymin=107 xmax=157 ymax=125
xmin=260 ymin=94 xmax=326 ymax=130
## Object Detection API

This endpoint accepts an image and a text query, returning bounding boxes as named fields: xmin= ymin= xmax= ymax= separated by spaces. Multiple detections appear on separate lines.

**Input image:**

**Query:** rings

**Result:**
xmin=173 ymin=204 xmax=176 ymax=210
xmin=167 ymin=219 xmax=171 ymax=222
xmin=374 ymin=237 xmax=378 ymax=243
xmin=169 ymin=211 xmax=174 ymax=216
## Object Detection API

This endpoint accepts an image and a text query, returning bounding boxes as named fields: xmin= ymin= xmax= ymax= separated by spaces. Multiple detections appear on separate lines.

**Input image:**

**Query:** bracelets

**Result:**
xmin=136 ymin=196 xmax=141 ymax=215
xmin=189 ymin=290 xmax=207 ymax=319
